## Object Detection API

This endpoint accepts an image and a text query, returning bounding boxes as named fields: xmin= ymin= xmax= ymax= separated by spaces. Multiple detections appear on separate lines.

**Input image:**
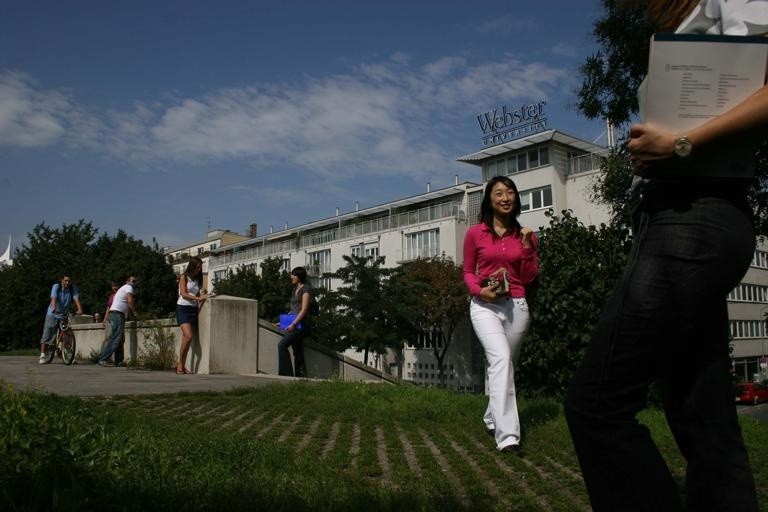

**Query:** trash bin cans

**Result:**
xmin=674 ymin=131 xmax=693 ymax=158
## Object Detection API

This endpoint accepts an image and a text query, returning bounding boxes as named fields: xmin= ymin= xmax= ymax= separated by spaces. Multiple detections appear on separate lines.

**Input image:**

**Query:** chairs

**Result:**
xmin=488 ymin=429 xmax=525 ymax=454
xmin=39 ymin=356 xmax=46 ymax=364
xmin=72 ymin=358 xmax=78 ymax=364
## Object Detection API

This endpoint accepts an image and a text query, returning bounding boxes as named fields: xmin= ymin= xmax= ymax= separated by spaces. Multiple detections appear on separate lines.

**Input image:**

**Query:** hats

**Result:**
xmin=735 ymin=383 xmax=768 ymax=404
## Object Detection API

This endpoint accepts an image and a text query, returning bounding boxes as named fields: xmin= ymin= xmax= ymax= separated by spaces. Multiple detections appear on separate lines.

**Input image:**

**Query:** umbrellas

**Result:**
xmin=98 ymin=359 xmax=126 ymax=367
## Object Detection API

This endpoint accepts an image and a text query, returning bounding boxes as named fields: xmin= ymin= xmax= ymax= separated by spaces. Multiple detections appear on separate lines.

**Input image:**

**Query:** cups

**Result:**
xmin=128 ymin=278 xmax=136 ymax=282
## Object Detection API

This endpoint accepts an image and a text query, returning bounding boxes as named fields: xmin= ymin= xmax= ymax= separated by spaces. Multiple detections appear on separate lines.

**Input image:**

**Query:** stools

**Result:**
xmin=109 ymin=309 xmax=125 ymax=316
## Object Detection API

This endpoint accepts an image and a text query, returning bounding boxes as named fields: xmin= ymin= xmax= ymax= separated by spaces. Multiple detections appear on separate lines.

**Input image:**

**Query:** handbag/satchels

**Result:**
xmin=292 ymin=321 xmax=296 ymax=327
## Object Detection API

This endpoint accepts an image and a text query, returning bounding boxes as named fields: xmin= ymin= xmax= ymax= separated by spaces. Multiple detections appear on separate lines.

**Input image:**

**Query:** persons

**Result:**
xmin=272 ymin=266 xmax=318 ymax=378
xmin=102 ymin=276 xmax=128 ymax=365
xmin=96 ymin=273 xmax=144 ymax=368
xmin=94 ymin=313 xmax=102 ymax=323
xmin=37 ymin=274 xmax=83 ymax=365
xmin=174 ymin=257 xmax=215 ymax=375
xmin=462 ymin=175 xmax=539 ymax=452
xmin=555 ymin=0 xmax=768 ymax=512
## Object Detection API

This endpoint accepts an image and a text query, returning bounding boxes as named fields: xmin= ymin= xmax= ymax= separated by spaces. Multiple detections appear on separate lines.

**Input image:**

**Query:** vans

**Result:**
xmin=175 ymin=359 xmax=194 ymax=374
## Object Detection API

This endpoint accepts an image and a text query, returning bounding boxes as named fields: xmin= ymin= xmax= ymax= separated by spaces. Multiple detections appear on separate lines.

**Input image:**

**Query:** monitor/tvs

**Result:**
xmin=279 ymin=314 xmax=302 ymax=330
xmin=480 ymin=268 xmax=512 ymax=297
xmin=643 ymin=30 xmax=767 ymax=136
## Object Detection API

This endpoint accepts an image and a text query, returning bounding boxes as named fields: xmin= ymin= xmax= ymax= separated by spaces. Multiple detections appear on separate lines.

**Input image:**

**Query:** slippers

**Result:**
xmin=309 ymin=300 xmax=319 ymax=315
